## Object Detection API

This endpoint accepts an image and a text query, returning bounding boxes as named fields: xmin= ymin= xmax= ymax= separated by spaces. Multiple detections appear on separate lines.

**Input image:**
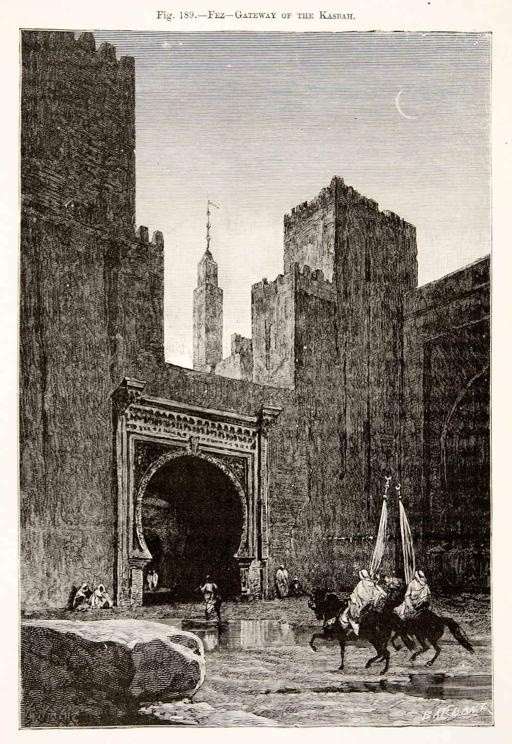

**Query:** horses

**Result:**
xmin=386 ymin=581 xmax=475 ymax=667
xmin=303 ymin=587 xmax=395 ymax=675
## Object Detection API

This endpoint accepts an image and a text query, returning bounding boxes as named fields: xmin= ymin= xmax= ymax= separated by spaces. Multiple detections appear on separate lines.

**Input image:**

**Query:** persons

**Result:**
xmin=146 ymin=570 xmax=153 ymax=590
xmin=394 ymin=570 xmax=430 ymax=621
xmin=200 ymin=575 xmax=218 ymax=620
xmin=90 ymin=584 xmax=113 ymax=609
xmin=73 ymin=583 xmax=92 ymax=611
xmin=275 ymin=564 xmax=289 ymax=598
xmin=340 ymin=570 xmax=380 ymax=635
xmin=153 ymin=570 xmax=159 ymax=589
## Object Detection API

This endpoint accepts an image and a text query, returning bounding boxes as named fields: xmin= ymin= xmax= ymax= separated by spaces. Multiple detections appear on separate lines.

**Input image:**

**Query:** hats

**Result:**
xmin=359 ymin=570 xmax=368 ymax=579
xmin=415 ymin=570 xmax=427 ymax=586
